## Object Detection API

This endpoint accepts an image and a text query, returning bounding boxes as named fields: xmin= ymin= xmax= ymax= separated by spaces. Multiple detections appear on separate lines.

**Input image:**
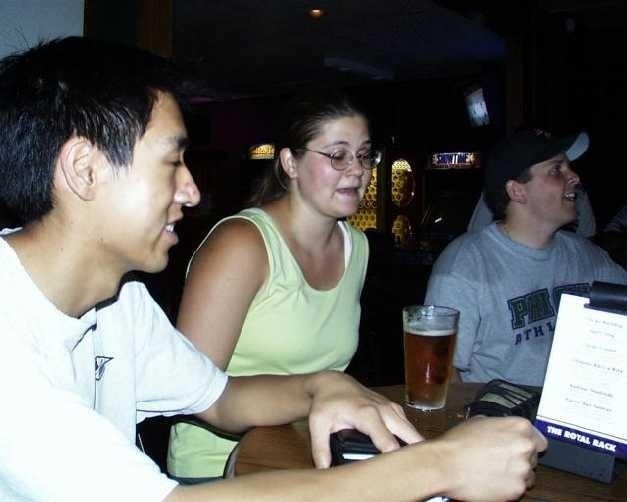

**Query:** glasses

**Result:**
xmin=306 ymin=148 xmax=382 ymax=171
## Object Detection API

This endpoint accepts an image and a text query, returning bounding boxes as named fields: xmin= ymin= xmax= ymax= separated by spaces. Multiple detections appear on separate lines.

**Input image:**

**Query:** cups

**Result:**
xmin=402 ymin=305 xmax=460 ymax=411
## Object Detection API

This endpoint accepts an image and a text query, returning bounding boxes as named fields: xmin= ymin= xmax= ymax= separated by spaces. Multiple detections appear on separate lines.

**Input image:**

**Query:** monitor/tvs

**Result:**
xmin=462 ymin=85 xmax=507 ymax=130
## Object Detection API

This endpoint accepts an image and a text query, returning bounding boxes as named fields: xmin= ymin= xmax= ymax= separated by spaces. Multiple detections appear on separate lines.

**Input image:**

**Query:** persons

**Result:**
xmin=423 ymin=124 xmax=627 ymax=389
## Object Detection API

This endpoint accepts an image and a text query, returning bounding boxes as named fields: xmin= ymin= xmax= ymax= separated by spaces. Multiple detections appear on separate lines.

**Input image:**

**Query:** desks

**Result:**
xmin=222 ymin=381 xmax=627 ymax=502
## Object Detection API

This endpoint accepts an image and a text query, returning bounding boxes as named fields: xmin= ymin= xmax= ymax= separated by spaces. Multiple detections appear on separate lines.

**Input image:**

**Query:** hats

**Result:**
xmin=484 ymin=131 xmax=589 ymax=184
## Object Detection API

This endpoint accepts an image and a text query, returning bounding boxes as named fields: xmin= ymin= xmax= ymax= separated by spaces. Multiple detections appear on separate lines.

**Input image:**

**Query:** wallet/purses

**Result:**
xmin=330 ymin=429 xmax=407 ymax=464
